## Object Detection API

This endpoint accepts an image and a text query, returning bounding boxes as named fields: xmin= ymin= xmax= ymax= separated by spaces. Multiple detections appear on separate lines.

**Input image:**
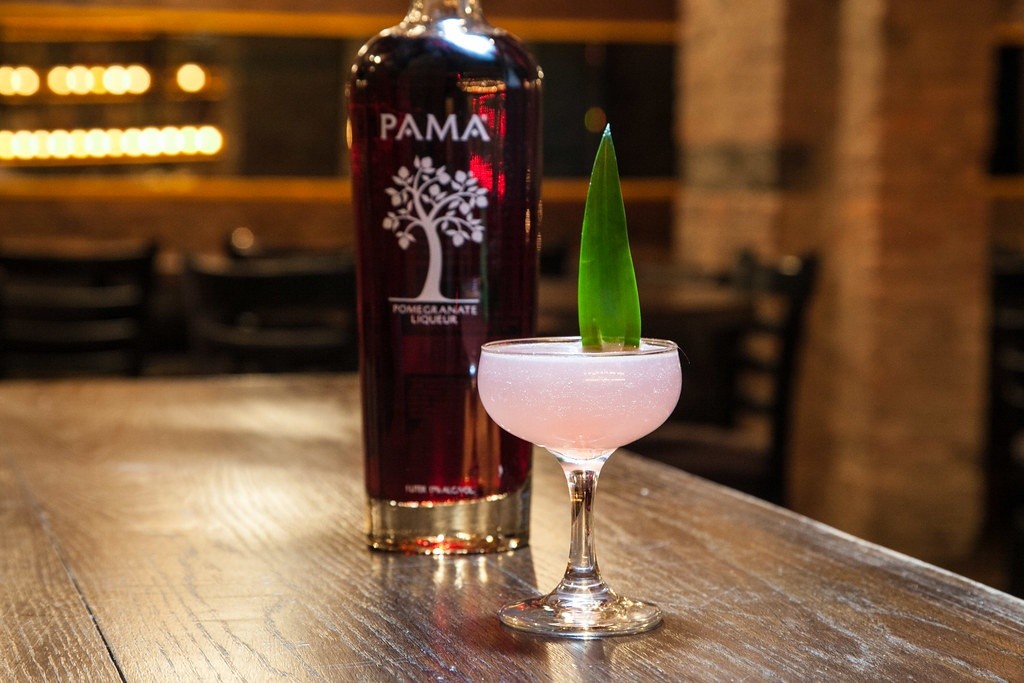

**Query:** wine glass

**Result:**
xmin=478 ymin=336 xmax=683 ymax=639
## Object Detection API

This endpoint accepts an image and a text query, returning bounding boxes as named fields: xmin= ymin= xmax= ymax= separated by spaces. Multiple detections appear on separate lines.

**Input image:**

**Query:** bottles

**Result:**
xmin=345 ymin=1 xmax=550 ymax=556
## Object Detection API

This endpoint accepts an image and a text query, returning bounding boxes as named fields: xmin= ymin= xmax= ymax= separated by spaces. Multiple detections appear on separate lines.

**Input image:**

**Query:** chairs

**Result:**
xmin=0 ymin=230 xmax=824 ymax=510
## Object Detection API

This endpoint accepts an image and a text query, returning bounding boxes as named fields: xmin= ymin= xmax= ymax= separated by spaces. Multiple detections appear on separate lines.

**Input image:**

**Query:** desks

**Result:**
xmin=1 ymin=370 xmax=1024 ymax=683
